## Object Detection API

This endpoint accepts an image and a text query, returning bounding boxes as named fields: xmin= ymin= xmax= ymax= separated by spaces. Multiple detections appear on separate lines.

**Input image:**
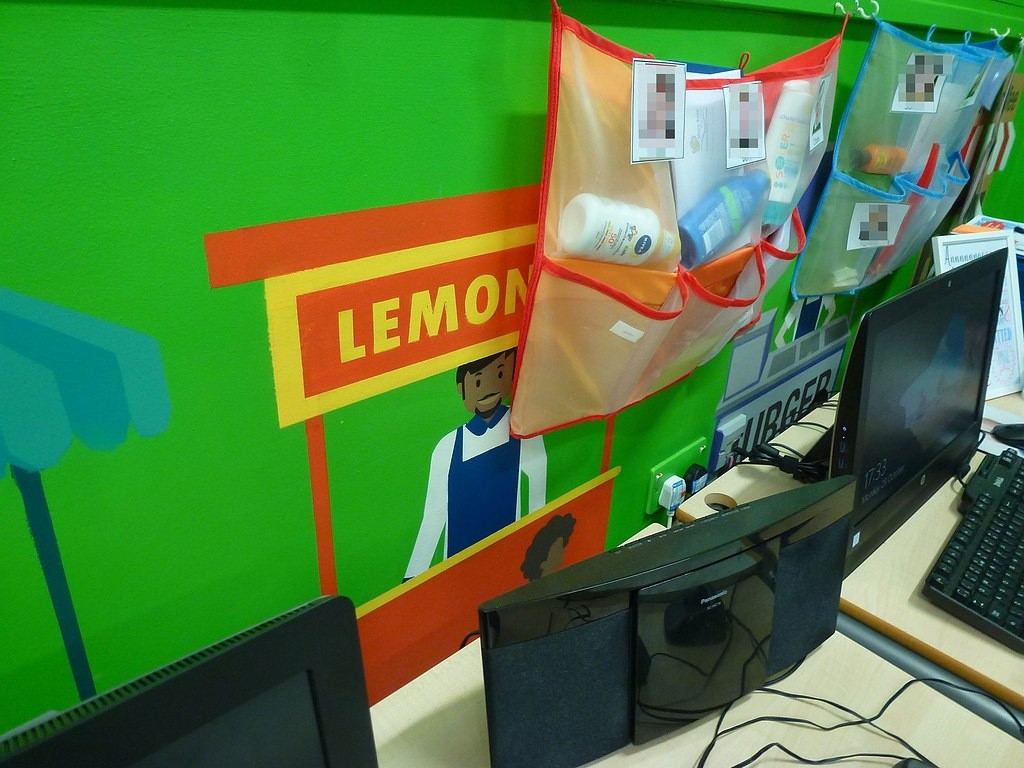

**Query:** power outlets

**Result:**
xmin=645 ymin=436 xmax=707 ymax=515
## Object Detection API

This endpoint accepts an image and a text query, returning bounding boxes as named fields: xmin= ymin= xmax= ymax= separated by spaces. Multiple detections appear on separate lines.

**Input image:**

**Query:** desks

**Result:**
xmin=677 ymin=391 xmax=1024 ymax=715
xmin=370 ymin=522 xmax=1024 ymax=768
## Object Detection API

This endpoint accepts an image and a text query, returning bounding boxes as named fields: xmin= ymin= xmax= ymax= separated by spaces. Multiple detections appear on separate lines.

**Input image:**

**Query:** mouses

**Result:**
xmin=993 ymin=424 xmax=1024 ymax=450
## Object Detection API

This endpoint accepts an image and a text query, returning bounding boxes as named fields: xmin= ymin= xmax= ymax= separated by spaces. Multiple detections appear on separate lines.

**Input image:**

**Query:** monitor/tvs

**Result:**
xmin=474 ymin=476 xmax=856 ymax=768
xmin=827 ymin=247 xmax=1011 ymax=578
xmin=0 ymin=593 xmax=377 ymax=768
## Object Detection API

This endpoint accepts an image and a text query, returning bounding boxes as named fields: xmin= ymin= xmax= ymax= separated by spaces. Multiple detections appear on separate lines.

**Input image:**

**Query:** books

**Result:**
xmin=932 ymin=231 xmax=1024 ymax=402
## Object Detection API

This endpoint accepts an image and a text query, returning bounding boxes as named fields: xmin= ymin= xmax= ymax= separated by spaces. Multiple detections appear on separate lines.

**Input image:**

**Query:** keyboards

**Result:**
xmin=922 ymin=449 xmax=1024 ymax=655
